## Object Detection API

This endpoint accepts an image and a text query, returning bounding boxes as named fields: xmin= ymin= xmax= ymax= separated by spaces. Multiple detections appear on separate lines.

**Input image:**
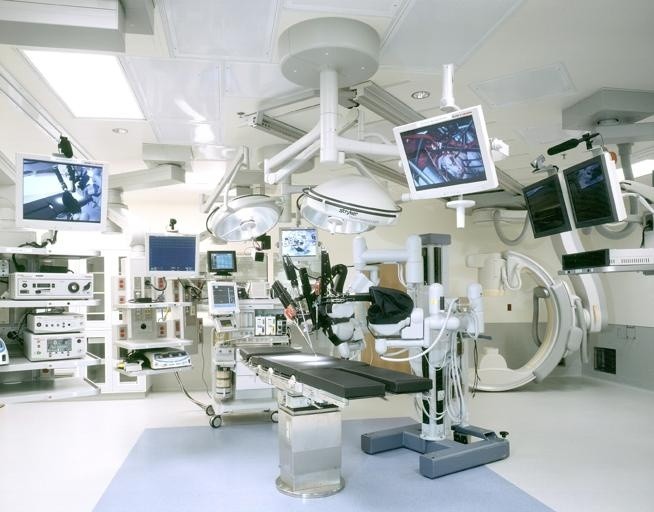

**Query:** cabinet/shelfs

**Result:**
xmin=0 ymin=246 xmax=101 ymax=404
xmin=113 ymin=302 xmax=193 ymax=378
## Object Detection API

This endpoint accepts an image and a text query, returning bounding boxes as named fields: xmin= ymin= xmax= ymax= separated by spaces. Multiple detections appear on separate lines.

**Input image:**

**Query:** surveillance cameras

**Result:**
xmin=531 ymin=155 xmax=546 ymax=168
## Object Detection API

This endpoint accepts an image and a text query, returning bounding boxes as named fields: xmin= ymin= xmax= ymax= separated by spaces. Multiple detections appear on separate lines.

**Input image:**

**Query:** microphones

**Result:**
xmin=549 ymin=134 xmax=600 ymax=156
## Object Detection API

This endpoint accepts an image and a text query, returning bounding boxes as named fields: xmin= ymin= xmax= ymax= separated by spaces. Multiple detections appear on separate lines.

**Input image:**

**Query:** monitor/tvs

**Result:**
xmin=16 ymin=151 xmax=109 ymax=233
xmin=520 ymin=174 xmax=573 ymax=240
xmin=208 ymin=281 xmax=240 ymax=313
xmin=145 ymin=233 xmax=201 ymax=279
xmin=562 ymin=152 xmax=627 ymax=229
xmin=392 ymin=105 xmax=498 ymax=201
xmin=207 ymin=250 xmax=237 ymax=276
xmin=279 ymin=226 xmax=320 ymax=261
